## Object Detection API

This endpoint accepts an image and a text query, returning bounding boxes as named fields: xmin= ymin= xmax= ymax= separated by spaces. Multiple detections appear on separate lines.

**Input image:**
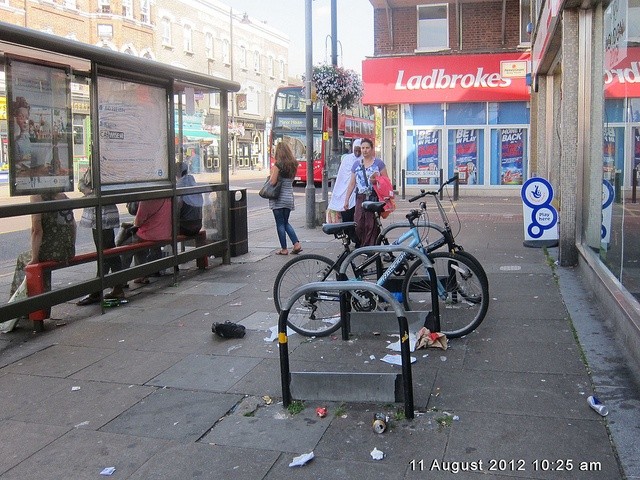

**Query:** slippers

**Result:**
xmin=290 ymin=248 xmax=303 ymax=254
xmin=275 ymin=250 xmax=288 ymax=255
xmin=76 ymin=295 xmax=101 ymax=306
xmin=104 ymin=291 xmax=124 ymax=299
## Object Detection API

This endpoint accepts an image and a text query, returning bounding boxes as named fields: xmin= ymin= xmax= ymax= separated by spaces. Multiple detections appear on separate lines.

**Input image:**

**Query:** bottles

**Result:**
xmin=102 ymin=297 xmax=130 ymax=309
xmin=586 ymin=395 xmax=608 ymax=417
xmin=371 ymin=413 xmax=386 ymax=433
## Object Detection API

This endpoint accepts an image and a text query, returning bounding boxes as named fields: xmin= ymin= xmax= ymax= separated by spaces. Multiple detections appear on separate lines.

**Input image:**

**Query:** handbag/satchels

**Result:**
xmin=259 ymin=170 xmax=282 ymax=199
xmin=126 ymin=199 xmax=166 ymax=235
xmin=361 ymin=160 xmax=385 ymax=211
xmin=115 ymin=222 xmax=134 ymax=248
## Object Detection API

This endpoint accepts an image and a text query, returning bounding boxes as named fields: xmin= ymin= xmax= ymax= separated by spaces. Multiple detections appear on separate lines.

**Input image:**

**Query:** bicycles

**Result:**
xmin=273 ymin=201 xmax=490 ymax=338
xmin=360 ymin=175 xmax=489 ymax=304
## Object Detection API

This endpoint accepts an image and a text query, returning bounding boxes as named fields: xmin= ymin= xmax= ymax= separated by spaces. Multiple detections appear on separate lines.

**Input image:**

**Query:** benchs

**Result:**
xmin=23 ymin=229 xmax=209 ymax=333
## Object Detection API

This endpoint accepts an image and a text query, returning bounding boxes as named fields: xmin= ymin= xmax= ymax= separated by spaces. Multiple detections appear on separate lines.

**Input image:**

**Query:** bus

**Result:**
xmin=270 ymin=85 xmax=376 ymax=186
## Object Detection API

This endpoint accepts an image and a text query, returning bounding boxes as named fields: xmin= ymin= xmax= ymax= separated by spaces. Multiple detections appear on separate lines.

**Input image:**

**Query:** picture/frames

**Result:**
xmin=4 ymin=51 xmax=76 ymax=195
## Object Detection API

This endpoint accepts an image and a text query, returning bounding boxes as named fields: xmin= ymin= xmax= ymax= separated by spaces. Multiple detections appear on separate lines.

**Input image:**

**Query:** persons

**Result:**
xmin=268 ymin=142 xmax=304 ymax=256
xmin=76 ymin=143 xmax=125 ymax=306
xmin=12 ymin=96 xmax=32 ymax=160
xmin=52 ymin=116 xmax=65 ymax=136
xmin=10 ymin=192 xmax=78 ymax=321
xmin=328 ymin=139 xmax=363 ymax=249
xmin=120 ymin=197 xmax=173 ymax=289
xmin=29 ymin=120 xmax=39 ymax=140
xmin=174 ymin=161 xmax=203 ymax=237
xmin=343 ymin=138 xmax=389 ymax=257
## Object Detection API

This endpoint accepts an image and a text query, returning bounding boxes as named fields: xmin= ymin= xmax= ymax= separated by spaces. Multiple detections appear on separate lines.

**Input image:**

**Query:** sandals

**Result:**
xmin=135 ymin=278 xmax=149 ymax=283
xmin=122 ymin=285 xmax=129 ymax=288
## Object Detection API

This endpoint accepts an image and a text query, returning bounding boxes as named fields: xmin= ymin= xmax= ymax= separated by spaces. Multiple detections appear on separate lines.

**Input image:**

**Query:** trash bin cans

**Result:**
xmin=230 ymin=189 xmax=248 ymax=256
